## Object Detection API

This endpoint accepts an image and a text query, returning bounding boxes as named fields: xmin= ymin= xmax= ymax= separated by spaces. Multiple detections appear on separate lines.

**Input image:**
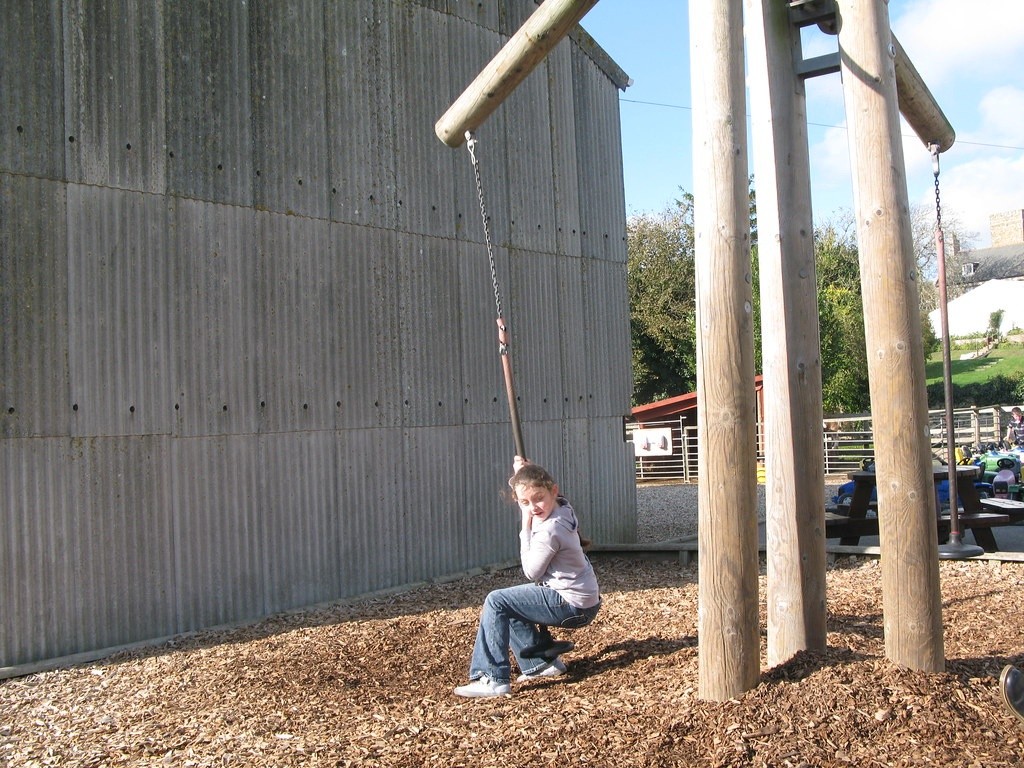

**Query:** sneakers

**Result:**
xmin=516 ymin=655 xmax=567 ymax=683
xmin=453 ymin=675 xmax=510 ymax=697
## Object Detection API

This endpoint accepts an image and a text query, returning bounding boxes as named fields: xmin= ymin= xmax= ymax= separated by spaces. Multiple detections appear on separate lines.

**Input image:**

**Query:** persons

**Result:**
xmin=454 ymin=455 xmax=603 ymax=696
xmin=1007 ymin=406 xmax=1024 ymax=452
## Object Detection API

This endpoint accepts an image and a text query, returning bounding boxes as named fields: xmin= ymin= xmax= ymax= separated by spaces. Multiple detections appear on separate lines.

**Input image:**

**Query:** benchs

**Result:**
xmin=824 ymin=501 xmax=1009 ymax=539
xmin=981 ymin=497 xmax=1024 ymax=515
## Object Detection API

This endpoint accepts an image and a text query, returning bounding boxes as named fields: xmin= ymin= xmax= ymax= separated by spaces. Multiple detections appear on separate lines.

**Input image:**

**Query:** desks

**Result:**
xmin=839 ymin=465 xmax=1000 ymax=552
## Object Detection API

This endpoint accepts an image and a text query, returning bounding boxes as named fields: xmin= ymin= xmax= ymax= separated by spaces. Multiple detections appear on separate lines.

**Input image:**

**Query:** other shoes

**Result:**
xmin=999 ymin=665 xmax=1024 ymax=725
